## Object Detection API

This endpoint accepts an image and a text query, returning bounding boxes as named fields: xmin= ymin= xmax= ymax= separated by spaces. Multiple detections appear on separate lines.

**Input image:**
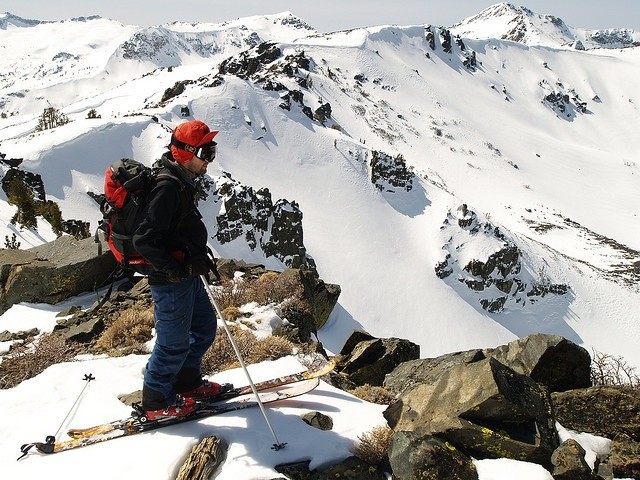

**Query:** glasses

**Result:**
xmin=171 ymin=134 xmax=218 ymax=162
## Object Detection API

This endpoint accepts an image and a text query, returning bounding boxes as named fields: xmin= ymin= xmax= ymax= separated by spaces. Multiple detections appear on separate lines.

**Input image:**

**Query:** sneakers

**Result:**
xmin=143 ymin=394 xmax=202 ymax=423
xmin=181 ymin=379 xmax=234 ymax=400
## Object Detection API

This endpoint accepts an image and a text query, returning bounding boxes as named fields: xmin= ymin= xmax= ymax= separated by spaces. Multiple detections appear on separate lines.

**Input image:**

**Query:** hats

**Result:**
xmin=171 ymin=121 xmax=219 ymax=163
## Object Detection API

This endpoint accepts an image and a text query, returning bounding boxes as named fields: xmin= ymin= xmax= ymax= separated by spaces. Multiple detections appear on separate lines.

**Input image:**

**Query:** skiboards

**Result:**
xmin=34 ymin=359 xmax=336 ymax=453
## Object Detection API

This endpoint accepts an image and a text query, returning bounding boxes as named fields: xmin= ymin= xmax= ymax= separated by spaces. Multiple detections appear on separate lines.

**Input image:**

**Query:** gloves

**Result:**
xmin=182 ymin=254 xmax=213 ymax=276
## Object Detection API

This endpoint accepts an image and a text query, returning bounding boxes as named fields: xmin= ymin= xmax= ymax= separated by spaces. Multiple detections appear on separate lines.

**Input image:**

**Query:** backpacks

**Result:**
xmin=95 ymin=159 xmax=189 ymax=313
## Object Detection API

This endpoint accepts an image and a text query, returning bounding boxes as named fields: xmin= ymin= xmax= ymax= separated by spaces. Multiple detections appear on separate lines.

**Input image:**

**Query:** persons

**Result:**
xmin=131 ymin=119 xmax=224 ymax=421
